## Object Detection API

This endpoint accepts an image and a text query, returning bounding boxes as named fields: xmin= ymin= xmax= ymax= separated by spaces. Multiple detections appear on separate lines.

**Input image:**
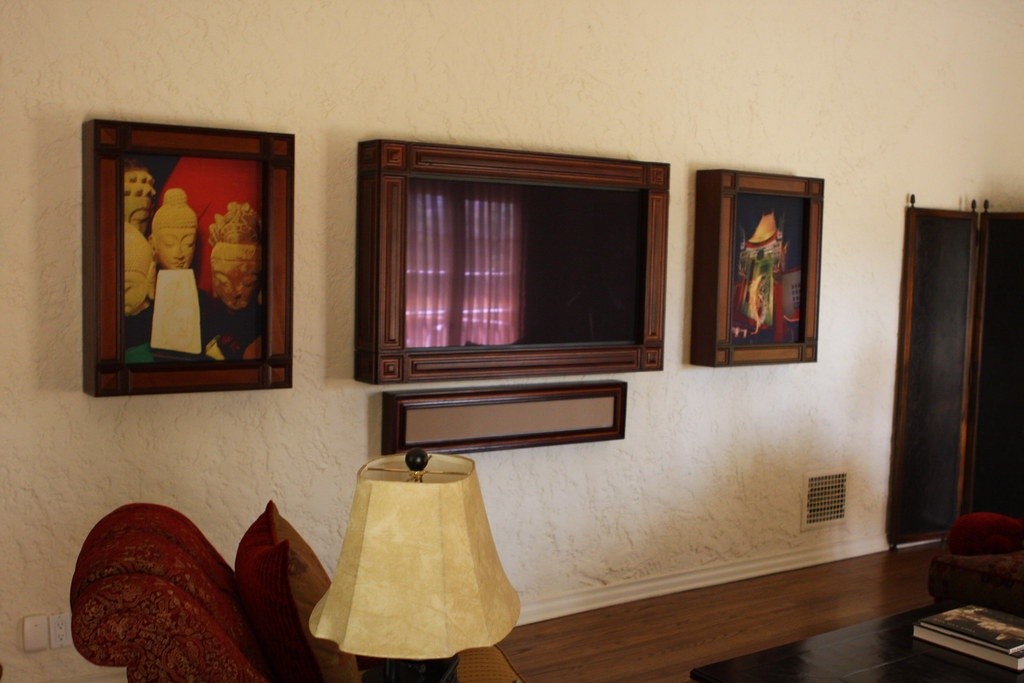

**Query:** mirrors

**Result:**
xmin=352 ymin=139 xmax=672 ymax=386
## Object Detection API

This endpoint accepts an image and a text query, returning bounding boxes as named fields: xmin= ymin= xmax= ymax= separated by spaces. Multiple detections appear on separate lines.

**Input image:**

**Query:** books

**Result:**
xmin=919 ymin=604 xmax=1024 ymax=654
xmin=913 ymin=621 xmax=1024 ymax=673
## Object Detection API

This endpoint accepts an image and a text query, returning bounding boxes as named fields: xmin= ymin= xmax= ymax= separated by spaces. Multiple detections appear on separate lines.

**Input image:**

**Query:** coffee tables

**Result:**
xmin=690 ymin=598 xmax=1024 ymax=683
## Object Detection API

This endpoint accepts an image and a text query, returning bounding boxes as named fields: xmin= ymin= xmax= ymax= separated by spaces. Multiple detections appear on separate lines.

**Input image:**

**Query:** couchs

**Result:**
xmin=66 ymin=501 xmax=523 ymax=682
xmin=929 ymin=512 xmax=1024 ymax=618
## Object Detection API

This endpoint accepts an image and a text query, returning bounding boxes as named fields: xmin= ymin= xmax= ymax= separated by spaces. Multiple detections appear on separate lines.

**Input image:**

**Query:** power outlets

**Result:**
xmin=49 ymin=613 xmax=72 ymax=649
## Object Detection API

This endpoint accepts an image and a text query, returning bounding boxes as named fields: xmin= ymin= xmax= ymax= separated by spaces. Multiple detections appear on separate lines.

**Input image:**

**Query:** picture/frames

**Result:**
xmin=82 ymin=119 xmax=295 ymax=399
xmin=691 ymin=168 xmax=827 ymax=368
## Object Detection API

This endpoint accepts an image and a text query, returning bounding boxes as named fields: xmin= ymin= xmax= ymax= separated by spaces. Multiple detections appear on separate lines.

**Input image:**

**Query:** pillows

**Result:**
xmin=235 ymin=499 xmax=363 ymax=683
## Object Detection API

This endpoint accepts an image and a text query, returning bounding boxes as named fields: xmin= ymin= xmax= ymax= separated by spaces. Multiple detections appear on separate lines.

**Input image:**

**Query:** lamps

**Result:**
xmin=308 ymin=448 xmax=522 ymax=682
